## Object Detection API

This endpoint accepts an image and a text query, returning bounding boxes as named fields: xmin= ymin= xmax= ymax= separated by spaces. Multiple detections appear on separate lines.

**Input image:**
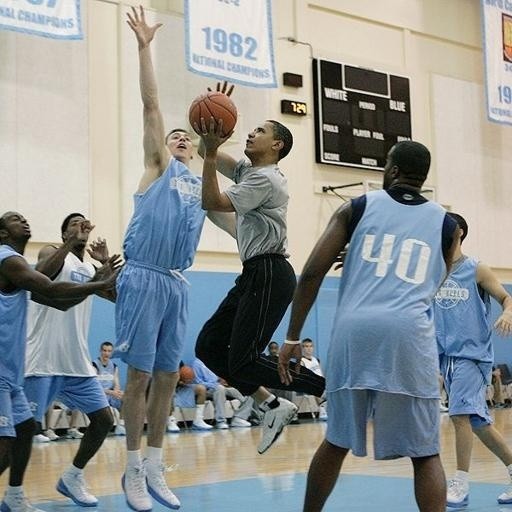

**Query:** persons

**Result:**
xmin=430 ymin=212 xmax=512 ymax=507
xmin=113 ymin=6 xmax=240 ymax=511
xmin=31 ymin=338 xmax=328 ymax=442
xmin=22 ymin=212 xmax=123 ymax=512
xmin=489 ymin=365 xmax=512 ymax=408
xmin=437 ymin=375 xmax=449 ymax=412
xmin=1 ymin=211 xmax=122 ymax=512
xmin=195 ymin=79 xmax=326 ymax=455
xmin=277 ymin=141 xmax=460 ymax=512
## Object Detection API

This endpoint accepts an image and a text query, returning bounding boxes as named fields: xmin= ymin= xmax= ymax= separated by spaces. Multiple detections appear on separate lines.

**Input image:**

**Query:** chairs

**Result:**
xmin=497 ymin=365 xmax=512 ymax=405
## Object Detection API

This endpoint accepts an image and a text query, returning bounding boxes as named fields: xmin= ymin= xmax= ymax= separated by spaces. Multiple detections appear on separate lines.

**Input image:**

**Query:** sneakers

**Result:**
xmin=145 ymin=464 xmax=181 ymax=510
xmin=444 ymin=478 xmax=470 ymax=508
xmin=192 ymin=420 xmax=214 ymax=430
xmin=0 ymin=490 xmax=45 ymax=512
xmin=166 ymin=421 xmax=180 ymax=432
xmin=42 ymin=429 xmax=60 ymax=441
xmin=216 ymin=421 xmax=228 ymax=428
xmin=256 ymin=397 xmax=298 ymax=455
xmin=65 ymin=429 xmax=85 ymax=439
xmin=439 ymin=405 xmax=448 ymax=412
xmin=494 ymin=404 xmax=507 ymax=408
xmin=121 ymin=465 xmax=153 ymax=512
xmin=115 ymin=425 xmax=126 ymax=435
xmin=55 ymin=473 xmax=99 ymax=507
xmin=231 ymin=418 xmax=252 ymax=426
xmin=497 ymin=485 xmax=512 ymax=504
xmin=32 ymin=434 xmax=50 ymax=442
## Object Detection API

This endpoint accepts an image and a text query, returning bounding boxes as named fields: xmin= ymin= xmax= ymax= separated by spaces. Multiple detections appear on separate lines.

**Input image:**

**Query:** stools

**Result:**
xmin=54 ymin=394 xmax=315 ymax=436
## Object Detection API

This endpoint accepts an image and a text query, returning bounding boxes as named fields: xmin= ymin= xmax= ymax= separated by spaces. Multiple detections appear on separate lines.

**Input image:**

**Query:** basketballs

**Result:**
xmin=189 ymin=92 xmax=237 ymax=137
xmin=179 ymin=367 xmax=195 ymax=380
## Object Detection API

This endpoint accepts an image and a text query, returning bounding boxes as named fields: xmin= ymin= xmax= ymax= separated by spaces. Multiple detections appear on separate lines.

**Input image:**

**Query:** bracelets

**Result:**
xmin=283 ymin=338 xmax=300 ymax=345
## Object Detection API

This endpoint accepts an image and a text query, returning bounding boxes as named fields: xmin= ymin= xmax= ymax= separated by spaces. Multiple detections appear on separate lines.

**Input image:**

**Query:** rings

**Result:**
xmin=201 ymin=132 xmax=208 ymax=137
